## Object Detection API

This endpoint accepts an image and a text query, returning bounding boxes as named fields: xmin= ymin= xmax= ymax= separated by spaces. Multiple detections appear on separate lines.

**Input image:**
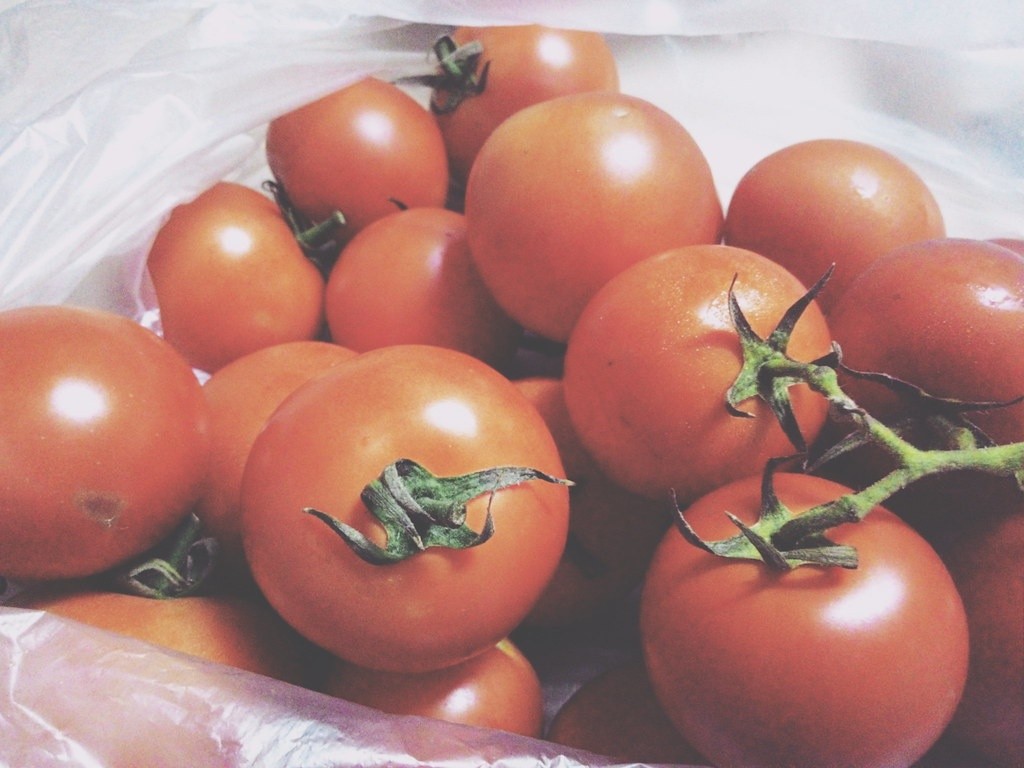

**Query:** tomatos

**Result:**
xmin=0 ymin=21 xmax=1024 ymax=768
